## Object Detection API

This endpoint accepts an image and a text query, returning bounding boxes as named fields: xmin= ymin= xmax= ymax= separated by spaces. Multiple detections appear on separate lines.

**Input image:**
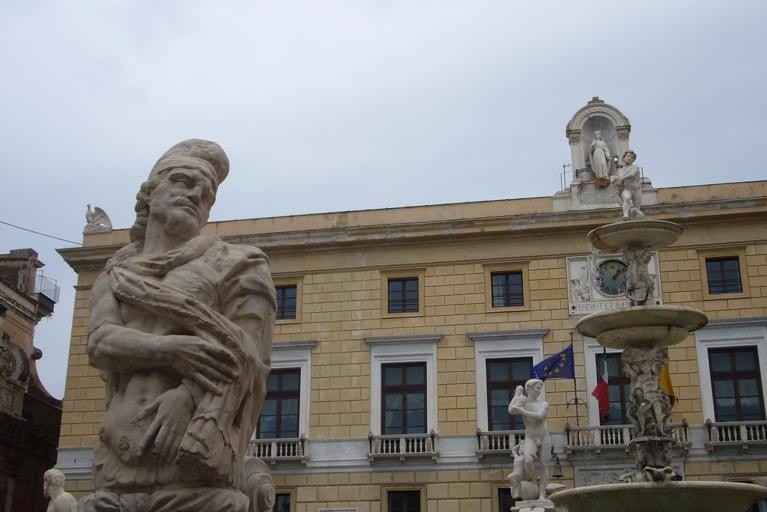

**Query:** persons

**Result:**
xmin=612 ymin=150 xmax=644 ymax=221
xmin=588 ymin=130 xmax=612 ymax=188
xmin=634 ymin=359 xmax=668 ymax=437
xmin=504 ymin=439 xmax=527 ymax=500
xmin=79 ymin=138 xmax=278 ymax=512
xmin=505 ymin=377 xmax=552 ymax=501
xmin=41 ymin=467 xmax=79 ymax=512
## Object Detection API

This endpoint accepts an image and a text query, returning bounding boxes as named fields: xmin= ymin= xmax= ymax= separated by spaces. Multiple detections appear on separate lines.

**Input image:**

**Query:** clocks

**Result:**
xmin=595 ymin=259 xmax=628 ymax=298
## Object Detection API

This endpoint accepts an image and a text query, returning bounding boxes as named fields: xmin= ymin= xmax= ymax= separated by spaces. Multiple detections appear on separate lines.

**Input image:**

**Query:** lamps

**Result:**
xmin=551 ymin=453 xmax=564 ymax=477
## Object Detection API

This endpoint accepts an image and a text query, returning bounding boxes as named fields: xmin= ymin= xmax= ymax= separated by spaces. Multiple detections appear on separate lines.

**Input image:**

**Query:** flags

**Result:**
xmin=590 ymin=352 xmax=609 ymax=419
xmin=530 ymin=344 xmax=574 ymax=383
